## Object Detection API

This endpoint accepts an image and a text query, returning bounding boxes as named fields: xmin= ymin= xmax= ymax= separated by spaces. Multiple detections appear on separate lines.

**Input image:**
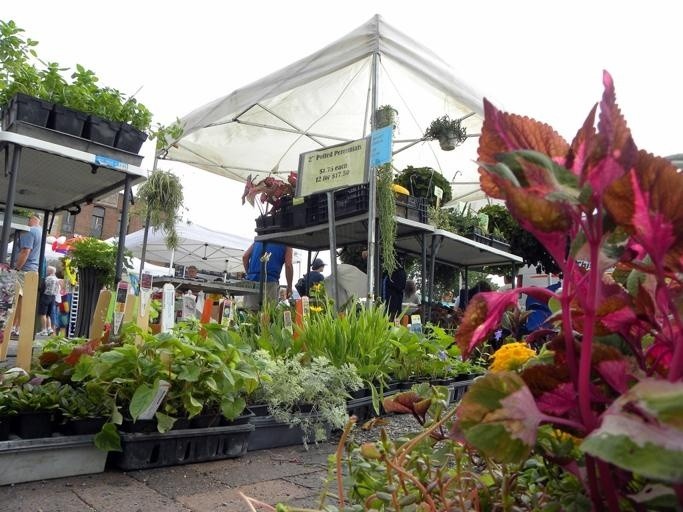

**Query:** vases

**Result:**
xmin=256 ymin=212 xmax=294 ymax=225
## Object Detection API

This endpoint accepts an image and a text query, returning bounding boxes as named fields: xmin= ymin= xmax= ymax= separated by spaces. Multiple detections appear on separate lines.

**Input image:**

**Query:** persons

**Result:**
xmin=10 ymin=212 xmax=43 ymax=334
xmin=297 ymin=258 xmax=326 ymax=296
xmin=279 ymin=287 xmax=288 ymax=301
xmin=402 ymin=280 xmax=421 ymax=304
xmin=381 ymin=261 xmax=406 ymax=320
xmin=440 ymin=290 xmax=454 ymax=306
xmin=242 ymin=240 xmax=293 ymax=314
xmin=497 ymin=274 xmax=513 ymax=293
xmin=38 ymin=265 xmax=63 ymax=337
xmin=187 ymin=266 xmax=199 ymax=279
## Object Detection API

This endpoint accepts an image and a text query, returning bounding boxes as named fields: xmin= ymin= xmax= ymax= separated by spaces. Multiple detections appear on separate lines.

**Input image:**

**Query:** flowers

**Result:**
xmin=253 ymin=348 xmax=364 ymax=452
xmin=403 ymin=321 xmax=486 ymax=386
xmin=241 ymin=171 xmax=297 ymax=215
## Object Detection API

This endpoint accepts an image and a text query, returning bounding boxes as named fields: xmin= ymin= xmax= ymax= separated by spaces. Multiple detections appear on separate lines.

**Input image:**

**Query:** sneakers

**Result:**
xmin=1 ymin=328 xmax=19 ymax=336
xmin=36 ymin=329 xmax=54 ymax=336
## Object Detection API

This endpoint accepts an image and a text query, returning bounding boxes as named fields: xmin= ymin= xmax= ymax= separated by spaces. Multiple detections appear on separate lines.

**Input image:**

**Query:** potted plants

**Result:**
xmin=64 ymin=236 xmax=134 ymax=339
xmin=1 ymin=373 xmax=124 ymax=485
xmin=73 ymin=323 xmax=274 ymax=471
xmin=291 ymin=304 xmax=400 ymax=424
xmin=0 ymin=20 xmax=153 ymax=154
xmin=370 ymin=105 xmax=399 ymax=139
xmin=374 ymin=165 xmax=519 ymax=282
xmin=129 ymin=169 xmax=184 ymax=251
xmin=421 ymin=95 xmax=468 ymax=151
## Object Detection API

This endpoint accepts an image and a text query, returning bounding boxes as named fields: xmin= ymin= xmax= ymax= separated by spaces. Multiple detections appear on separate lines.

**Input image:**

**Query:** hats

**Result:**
xmin=28 ymin=213 xmax=41 ymax=221
xmin=313 ymin=259 xmax=327 ymax=268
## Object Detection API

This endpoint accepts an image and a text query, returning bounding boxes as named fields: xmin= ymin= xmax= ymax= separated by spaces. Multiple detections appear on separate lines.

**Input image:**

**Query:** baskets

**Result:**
xmin=310 ymin=182 xmax=428 ymax=226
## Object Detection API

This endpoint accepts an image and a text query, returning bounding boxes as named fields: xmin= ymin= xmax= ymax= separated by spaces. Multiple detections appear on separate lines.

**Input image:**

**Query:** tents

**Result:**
xmin=113 ymin=219 xmax=302 ymax=285
